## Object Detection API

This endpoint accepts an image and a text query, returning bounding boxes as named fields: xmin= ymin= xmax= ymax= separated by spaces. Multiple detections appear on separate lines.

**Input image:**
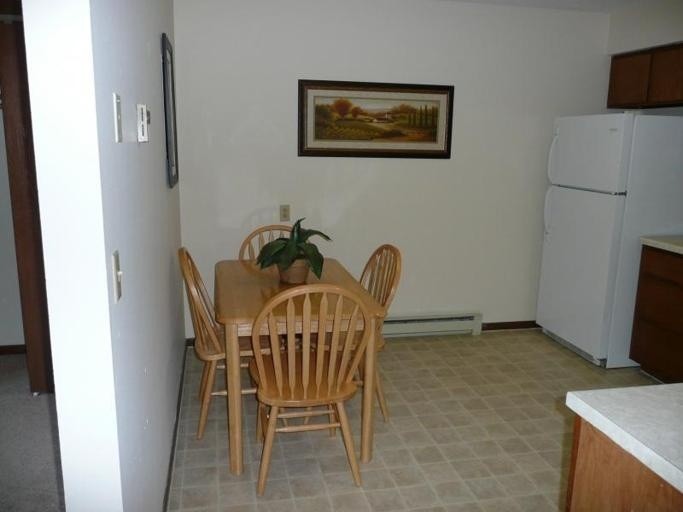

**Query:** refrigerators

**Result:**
xmin=532 ymin=114 xmax=683 ymax=369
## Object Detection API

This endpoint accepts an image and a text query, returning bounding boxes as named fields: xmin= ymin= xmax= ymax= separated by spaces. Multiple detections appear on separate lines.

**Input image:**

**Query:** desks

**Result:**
xmin=215 ymin=255 xmax=383 ymax=475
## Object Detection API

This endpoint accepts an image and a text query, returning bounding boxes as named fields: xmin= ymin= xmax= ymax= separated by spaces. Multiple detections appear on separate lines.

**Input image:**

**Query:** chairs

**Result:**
xmin=237 ymin=224 xmax=295 ymax=265
xmin=179 ymin=248 xmax=288 ymax=442
xmin=249 ymin=285 xmax=374 ymax=498
xmin=303 ymin=244 xmax=400 ymax=438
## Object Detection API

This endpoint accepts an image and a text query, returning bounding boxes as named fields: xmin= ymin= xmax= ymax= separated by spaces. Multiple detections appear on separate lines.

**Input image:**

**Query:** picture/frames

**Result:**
xmin=294 ymin=78 xmax=454 ymax=160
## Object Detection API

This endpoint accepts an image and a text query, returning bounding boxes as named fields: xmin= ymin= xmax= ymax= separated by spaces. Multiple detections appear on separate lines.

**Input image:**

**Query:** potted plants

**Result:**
xmin=255 ymin=217 xmax=333 ymax=284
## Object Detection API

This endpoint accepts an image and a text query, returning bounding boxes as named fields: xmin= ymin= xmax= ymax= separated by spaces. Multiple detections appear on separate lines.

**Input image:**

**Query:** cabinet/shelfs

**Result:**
xmin=629 ymin=246 xmax=681 ymax=382
xmin=607 ymin=39 xmax=682 ymax=110
